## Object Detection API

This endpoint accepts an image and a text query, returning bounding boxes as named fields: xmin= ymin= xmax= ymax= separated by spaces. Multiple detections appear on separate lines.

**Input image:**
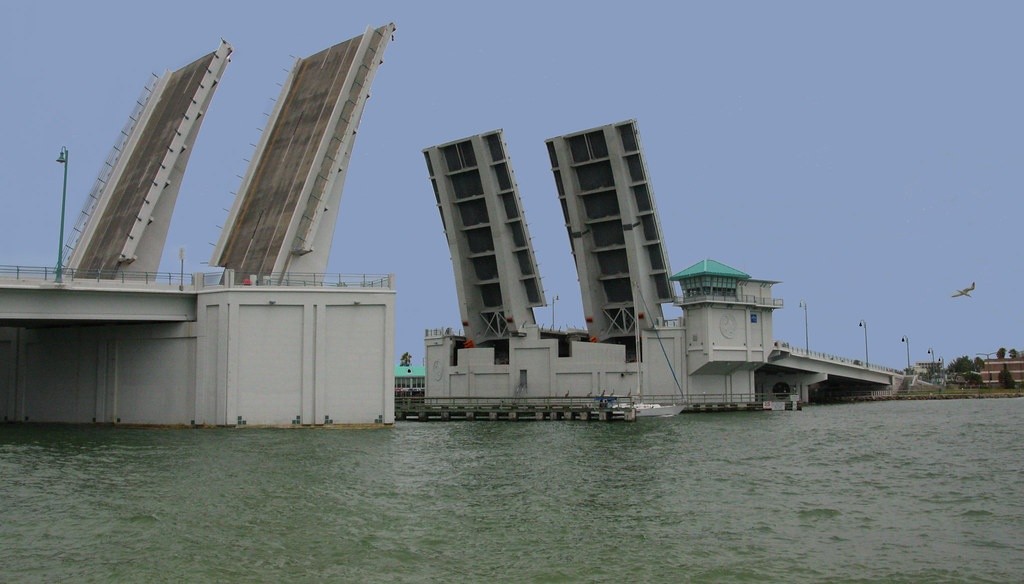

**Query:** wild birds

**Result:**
xmin=951 ymin=282 xmax=977 ymax=298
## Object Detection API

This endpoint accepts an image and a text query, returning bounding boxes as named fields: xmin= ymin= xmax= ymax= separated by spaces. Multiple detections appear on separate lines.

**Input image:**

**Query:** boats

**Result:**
xmin=604 ymin=402 xmax=688 ymax=419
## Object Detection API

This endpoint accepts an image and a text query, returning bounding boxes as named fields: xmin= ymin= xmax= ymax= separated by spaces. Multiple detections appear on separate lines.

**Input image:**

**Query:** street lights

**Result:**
xmin=859 ymin=320 xmax=869 ymax=367
xmin=798 ymin=302 xmax=810 ymax=356
xmin=55 ymin=144 xmax=70 ymax=283
xmin=975 ymin=352 xmax=998 ymax=393
xmin=901 ymin=335 xmax=910 ymax=368
xmin=927 ymin=347 xmax=935 ymax=371
xmin=939 ymin=356 xmax=944 ymax=375
